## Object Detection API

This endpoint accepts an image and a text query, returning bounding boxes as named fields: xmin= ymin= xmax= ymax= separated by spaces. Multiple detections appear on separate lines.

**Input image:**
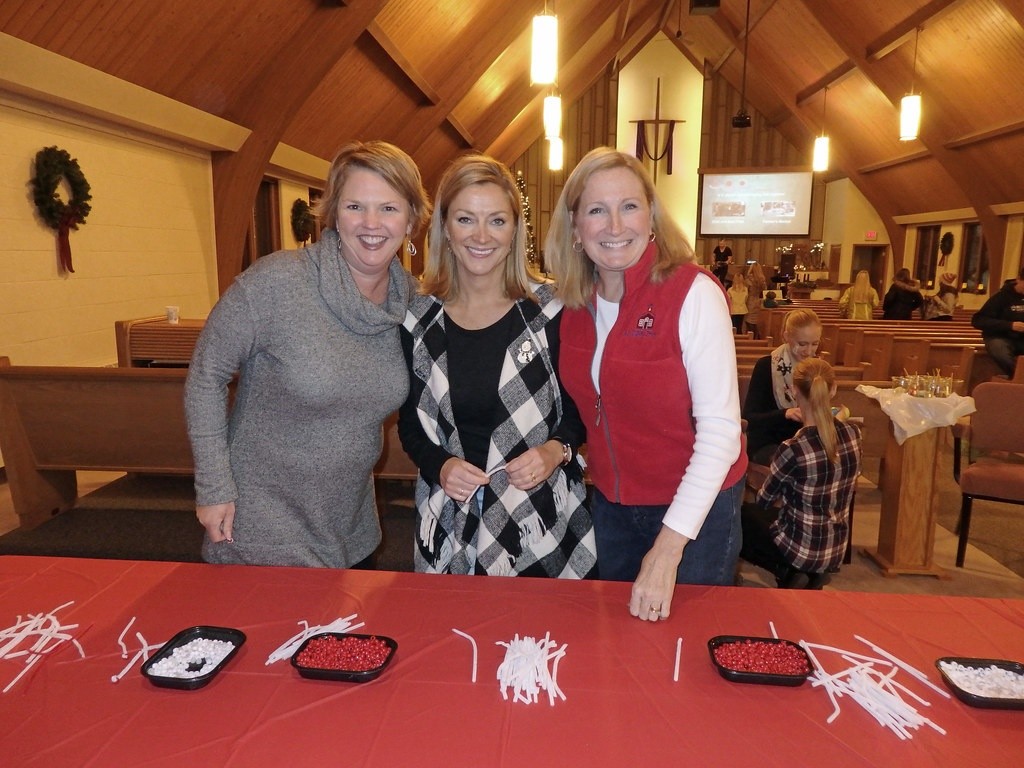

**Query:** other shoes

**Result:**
xmin=779 ymin=572 xmax=809 ymax=589
xmin=809 ymin=572 xmax=831 ymax=590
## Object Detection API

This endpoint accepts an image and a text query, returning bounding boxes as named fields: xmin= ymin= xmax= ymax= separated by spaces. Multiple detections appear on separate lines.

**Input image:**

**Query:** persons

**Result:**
xmin=542 ymin=147 xmax=749 ymax=621
xmin=740 ymin=359 xmax=862 ymax=590
xmin=396 ymin=154 xmax=599 ymax=580
xmin=972 ymin=269 xmax=1024 ymax=376
xmin=743 ymin=309 xmax=822 ymax=466
xmin=182 ymin=142 xmax=430 ymax=572
xmin=712 ymin=237 xmax=733 ymax=286
xmin=764 ymin=292 xmax=778 ymax=308
xmin=726 ymin=272 xmax=749 ymax=334
xmin=882 ymin=268 xmax=923 ymax=320
xmin=923 ymin=273 xmax=958 ymax=321
xmin=839 ymin=270 xmax=879 ymax=320
xmin=744 ymin=261 xmax=767 ymax=339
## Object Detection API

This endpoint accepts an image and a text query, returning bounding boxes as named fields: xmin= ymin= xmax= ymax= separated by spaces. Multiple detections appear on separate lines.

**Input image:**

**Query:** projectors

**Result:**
xmin=731 ymin=115 xmax=752 ymax=128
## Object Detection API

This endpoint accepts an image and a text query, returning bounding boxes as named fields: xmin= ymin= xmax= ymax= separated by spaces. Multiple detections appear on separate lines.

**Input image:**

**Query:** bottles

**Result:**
xmin=934 ymin=656 xmax=1024 ymax=710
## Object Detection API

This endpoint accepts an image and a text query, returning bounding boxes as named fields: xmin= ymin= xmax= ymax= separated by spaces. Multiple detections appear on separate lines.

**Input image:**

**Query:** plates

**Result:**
xmin=289 ymin=632 xmax=399 ymax=683
xmin=707 ymin=634 xmax=814 ymax=687
xmin=140 ymin=624 xmax=247 ymax=692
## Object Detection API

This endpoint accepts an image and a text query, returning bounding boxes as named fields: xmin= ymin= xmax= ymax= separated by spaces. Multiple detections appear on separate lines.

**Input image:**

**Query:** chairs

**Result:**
xmin=950 ymin=378 xmax=1024 ymax=570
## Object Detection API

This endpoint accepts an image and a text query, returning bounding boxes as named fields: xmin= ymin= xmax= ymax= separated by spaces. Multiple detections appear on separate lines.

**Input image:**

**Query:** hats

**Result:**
xmin=940 ymin=272 xmax=958 ymax=286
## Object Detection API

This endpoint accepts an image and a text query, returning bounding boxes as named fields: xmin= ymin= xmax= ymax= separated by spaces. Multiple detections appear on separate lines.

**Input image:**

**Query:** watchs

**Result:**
xmin=551 ymin=437 xmax=572 ymax=465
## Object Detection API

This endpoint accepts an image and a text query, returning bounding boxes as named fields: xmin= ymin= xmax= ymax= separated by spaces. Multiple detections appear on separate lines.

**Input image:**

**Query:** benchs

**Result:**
xmin=2 ymin=302 xmax=1024 ymax=588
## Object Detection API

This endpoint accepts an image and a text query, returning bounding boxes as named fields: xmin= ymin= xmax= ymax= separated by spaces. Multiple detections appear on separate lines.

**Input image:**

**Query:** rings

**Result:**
xmin=530 ymin=473 xmax=536 ymax=482
xmin=650 ymin=607 xmax=660 ymax=613
xmin=460 ymin=489 xmax=463 ymax=495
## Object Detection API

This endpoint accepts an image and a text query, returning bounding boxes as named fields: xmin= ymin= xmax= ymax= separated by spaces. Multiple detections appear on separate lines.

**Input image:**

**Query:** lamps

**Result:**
xmin=542 ymin=90 xmax=562 ymax=141
xmin=547 ymin=139 xmax=566 ymax=172
xmin=814 ymin=78 xmax=831 ymax=173
xmin=899 ymin=20 xmax=932 ymax=141
xmin=530 ymin=0 xmax=563 ymax=86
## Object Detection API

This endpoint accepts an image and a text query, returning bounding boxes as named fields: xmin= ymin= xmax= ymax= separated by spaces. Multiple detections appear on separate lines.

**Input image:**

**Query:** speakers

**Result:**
xmin=781 ymin=253 xmax=796 ymax=278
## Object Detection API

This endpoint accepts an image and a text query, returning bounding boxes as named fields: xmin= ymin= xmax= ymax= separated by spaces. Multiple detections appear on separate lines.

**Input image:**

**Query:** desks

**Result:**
xmin=1 ymin=555 xmax=1024 ymax=768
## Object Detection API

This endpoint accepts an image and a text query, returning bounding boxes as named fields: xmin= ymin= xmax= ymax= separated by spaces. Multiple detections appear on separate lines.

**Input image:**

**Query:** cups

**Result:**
xmin=892 ymin=374 xmax=953 ymax=398
xmin=166 ymin=306 xmax=179 ymax=325
